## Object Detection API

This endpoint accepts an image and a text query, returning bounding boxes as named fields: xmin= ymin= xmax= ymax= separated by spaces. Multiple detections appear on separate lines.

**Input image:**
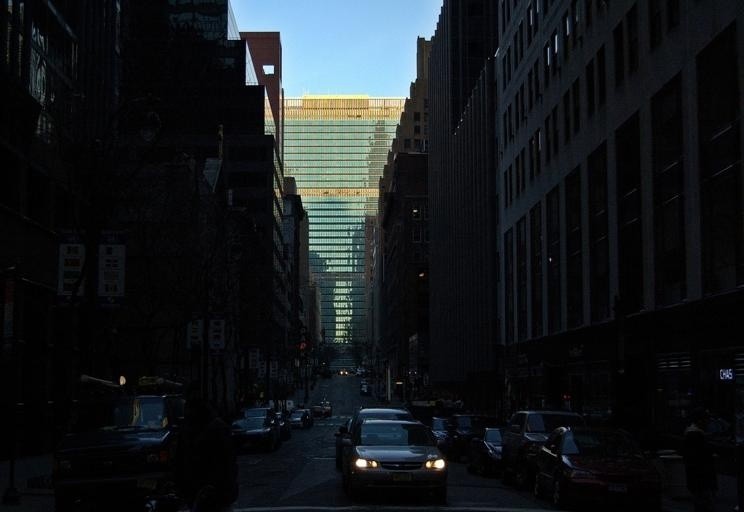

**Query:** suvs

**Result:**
xmin=502 ymin=409 xmax=588 ymax=484
xmin=50 ymin=393 xmax=233 ymax=504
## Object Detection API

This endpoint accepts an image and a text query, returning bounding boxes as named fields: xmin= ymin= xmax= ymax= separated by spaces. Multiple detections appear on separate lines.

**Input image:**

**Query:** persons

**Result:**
xmin=680 ymin=402 xmax=726 ymax=511
xmin=185 ymin=399 xmax=243 ymax=511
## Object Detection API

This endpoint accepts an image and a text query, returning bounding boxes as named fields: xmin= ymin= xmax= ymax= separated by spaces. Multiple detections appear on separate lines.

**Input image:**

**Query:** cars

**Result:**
xmin=526 ymin=425 xmax=660 ymax=508
xmin=230 ymin=399 xmax=331 ymax=448
xmin=336 ymin=370 xmax=511 ymax=485
